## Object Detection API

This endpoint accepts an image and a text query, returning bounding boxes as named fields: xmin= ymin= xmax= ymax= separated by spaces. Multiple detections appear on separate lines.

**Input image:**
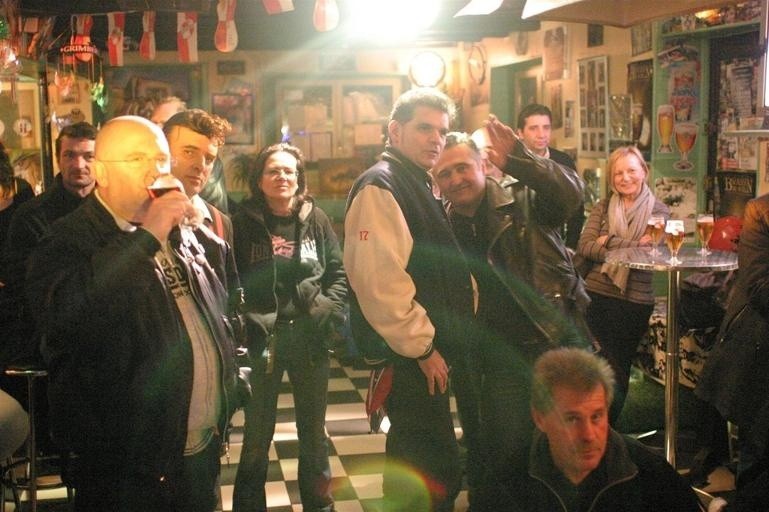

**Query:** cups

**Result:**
xmin=656 ymin=105 xmax=676 ymax=154
xmin=673 ymin=70 xmax=694 ymax=122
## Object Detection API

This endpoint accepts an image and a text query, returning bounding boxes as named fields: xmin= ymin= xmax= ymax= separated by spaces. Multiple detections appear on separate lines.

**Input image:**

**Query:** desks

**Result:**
xmin=605 ymin=247 xmax=739 ymax=472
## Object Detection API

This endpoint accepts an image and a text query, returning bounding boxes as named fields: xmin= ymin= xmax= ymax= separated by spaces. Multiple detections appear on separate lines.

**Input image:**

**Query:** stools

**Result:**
xmin=0 ymin=364 xmax=78 ymax=512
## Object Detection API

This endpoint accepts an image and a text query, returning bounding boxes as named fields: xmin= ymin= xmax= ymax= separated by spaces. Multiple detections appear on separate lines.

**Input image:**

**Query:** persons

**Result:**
xmin=704 ymin=193 xmax=769 ymax=511
xmin=472 ymin=126 xmax=497 ymax=176
xmin=518 ymin=105 xmax=584 ymax=249
xmin=227 ymin=144 xmax=347 ymax=511
xmin=433 ymin=114 xmax=589 ymax=467
xmin=0 ymin=87 xmax=252 ymax=510
xmin=341 ymin=85 xmax=471 ymax=511
xmin=577 ymin=146 xmax=669 ymax=425
xmin=466 ymin=347 xmax=704 ymax=511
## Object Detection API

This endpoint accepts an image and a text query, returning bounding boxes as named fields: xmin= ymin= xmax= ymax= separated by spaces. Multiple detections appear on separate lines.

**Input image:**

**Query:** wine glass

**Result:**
xmin=664 ymin=219 xmax=684 ymax=266
xmin=696 ymin=213 xmax=714 ymax=257
xmin=645 ymin=215 xmax=666 ymax=258
xmin=668 ymin=124 xmax=698 ymax=169
xmin=145 ymin=167 xmax=204 ymax=232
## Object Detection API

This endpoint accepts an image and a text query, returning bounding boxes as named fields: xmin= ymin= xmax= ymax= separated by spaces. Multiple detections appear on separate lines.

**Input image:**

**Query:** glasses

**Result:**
xmin=100 ymin=155 xmax=177 ymax=166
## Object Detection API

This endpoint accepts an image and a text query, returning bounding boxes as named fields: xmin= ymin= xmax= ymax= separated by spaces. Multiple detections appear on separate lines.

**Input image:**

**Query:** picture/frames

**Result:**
xmin=212 ymin=93 xmax=255 ymax=145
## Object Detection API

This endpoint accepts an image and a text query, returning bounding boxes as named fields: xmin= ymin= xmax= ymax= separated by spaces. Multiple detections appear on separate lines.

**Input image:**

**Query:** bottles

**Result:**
xmin=631 ymin=103 xmax=644 ymax=148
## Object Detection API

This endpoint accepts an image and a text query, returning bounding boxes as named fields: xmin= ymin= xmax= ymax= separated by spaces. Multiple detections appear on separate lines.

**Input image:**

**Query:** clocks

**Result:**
xmin=467 ymin=46 xmax=486 ymax=85
